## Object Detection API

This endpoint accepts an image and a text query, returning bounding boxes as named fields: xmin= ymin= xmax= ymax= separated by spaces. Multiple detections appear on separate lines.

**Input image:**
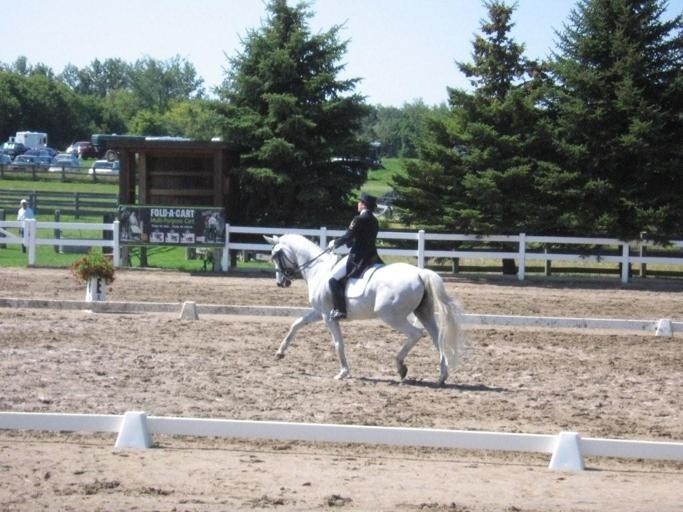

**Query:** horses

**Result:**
xmin=262 ymin=233 xmax=464 ymax=388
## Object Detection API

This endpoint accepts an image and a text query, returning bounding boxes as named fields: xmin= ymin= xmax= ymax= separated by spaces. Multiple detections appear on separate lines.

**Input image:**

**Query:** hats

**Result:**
xmin=356 ymin=192 xmax=378 ymax=208
xmin=20 ymin=200 xmax=26 ymax=204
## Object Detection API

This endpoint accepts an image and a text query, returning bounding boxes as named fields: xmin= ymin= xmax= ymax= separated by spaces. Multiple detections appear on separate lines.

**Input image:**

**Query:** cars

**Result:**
xmin=0 ymin=130 xmax=120 ymax=175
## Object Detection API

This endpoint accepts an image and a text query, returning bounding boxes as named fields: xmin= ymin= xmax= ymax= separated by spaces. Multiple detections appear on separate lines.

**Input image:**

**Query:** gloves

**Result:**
xmin=328 ymin=240 xmax=335 ymax=251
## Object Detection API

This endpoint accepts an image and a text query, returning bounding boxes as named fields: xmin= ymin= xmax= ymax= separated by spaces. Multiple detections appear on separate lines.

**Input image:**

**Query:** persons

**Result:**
xmin=325 ymin=192 xmax=383 ymax=319
xmin=14 ymin=199 xmax=34 ymax=254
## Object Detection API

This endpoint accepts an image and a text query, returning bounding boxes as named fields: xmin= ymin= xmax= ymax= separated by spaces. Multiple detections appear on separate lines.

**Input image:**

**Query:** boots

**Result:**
xmin=327 ymin=278 xmax=345 ymax=318
